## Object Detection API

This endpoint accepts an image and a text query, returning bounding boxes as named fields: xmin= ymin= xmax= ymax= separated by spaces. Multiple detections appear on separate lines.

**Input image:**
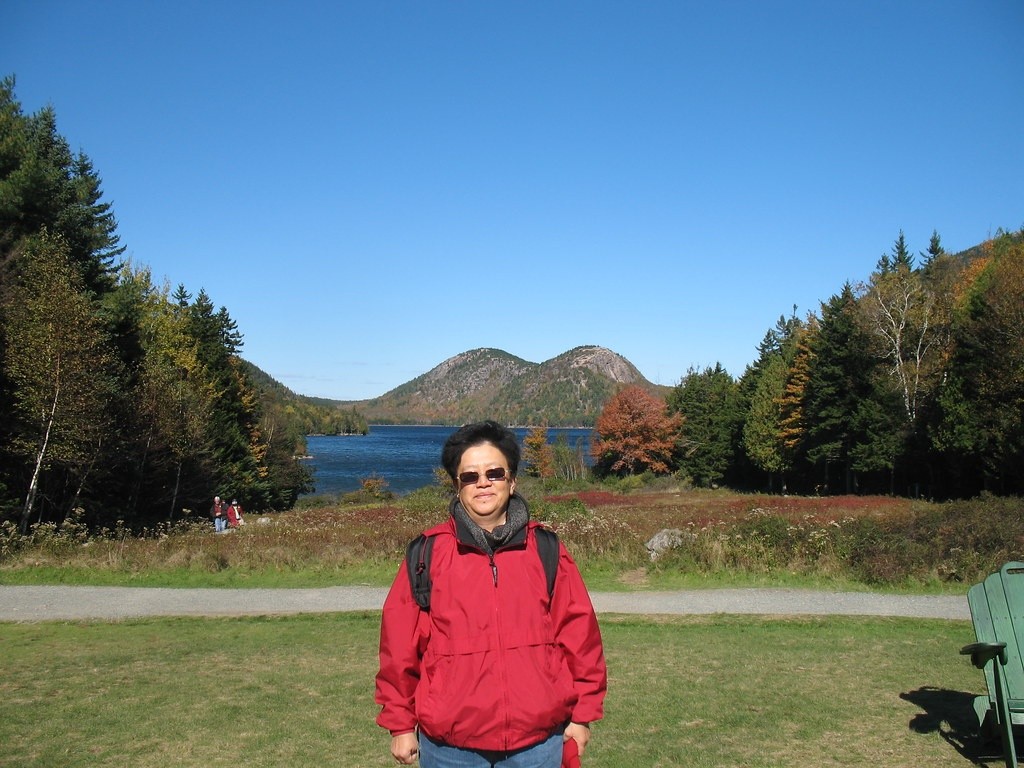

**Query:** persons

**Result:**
xmin=211 ymin=496 xmax=245 ymax=532
xmin=375 ymin=419 xmax=607 ymax=768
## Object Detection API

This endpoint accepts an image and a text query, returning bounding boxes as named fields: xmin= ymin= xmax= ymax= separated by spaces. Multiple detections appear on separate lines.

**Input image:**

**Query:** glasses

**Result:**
xmin=457 ymin=468 xmax=512 ymax=482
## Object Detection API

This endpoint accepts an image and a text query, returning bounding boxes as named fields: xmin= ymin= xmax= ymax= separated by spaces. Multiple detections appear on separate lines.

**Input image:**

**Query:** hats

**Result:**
xmin=232 ymin=501 xmax=238 ymax=504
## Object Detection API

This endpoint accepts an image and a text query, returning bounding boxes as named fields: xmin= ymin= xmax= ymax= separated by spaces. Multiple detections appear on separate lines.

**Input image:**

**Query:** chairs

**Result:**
xmin=959 ymin=561 xmax=1024 ymax=768
